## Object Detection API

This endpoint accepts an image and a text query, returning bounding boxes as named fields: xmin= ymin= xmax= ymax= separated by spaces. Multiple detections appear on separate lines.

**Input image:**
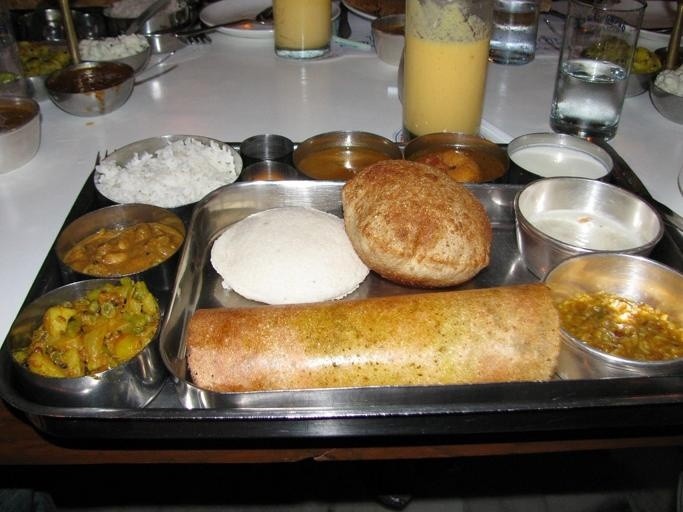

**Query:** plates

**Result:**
xmin=199 ymin=2 xmax=344 ymax=37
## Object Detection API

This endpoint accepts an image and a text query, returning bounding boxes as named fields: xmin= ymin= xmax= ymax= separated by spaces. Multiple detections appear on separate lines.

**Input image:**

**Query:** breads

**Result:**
xmin=342 ymin=156 xmax=494 ymax=288
xmin=187 ymin=283 xmax=563 ymax=393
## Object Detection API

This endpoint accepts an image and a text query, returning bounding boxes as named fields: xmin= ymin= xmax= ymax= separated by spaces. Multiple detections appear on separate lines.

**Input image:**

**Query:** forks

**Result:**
xmin=180 ymin=26 xmax=213 ymax=45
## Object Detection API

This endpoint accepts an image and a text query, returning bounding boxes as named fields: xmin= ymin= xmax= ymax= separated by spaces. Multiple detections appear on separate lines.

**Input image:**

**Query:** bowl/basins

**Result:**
xmin=543 ymin=253 xmax=683 ymax=380
xmin=6 ymin=278 xmax=168 ymax=410
xmin=54 ymin=204 xmax=186 ymax=293
xmin=514 ymin=177 xmax=665 ymax=278
xmin=291 ymin=129 xmax=403 ymax=181
xmin=403 ymin=132 xmax=508 ymax=184
xmin=573 ymin=40 xmax=662 ymax=95
xmin=370 ymin=14 xmax=405 ymax=68
xmin=507 ymin=132 xmax=615 ymax=184
xmin=0 ymin=35 xmax=156 ymax=174
xmin=648 ymin=80 xmax=683 ymax=125
xmin=92 ymin=134 xmax=244 ymax=222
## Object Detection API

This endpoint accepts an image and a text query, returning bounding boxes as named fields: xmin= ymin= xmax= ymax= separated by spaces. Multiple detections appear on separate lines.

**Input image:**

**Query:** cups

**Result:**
xmin=549 ymin=0 xmax=647 ymax=141
xmin=272 ymin=0 xmax=333 ymax=60
xmin=404 ymin=0 xmax=489 ymax=137
xmin=489 ymin=0 xmax=539 ymax=68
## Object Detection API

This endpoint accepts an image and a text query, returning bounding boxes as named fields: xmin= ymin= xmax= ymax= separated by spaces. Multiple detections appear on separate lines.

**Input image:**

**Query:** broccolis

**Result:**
xmin=79 ymin=32 xmax=149 ymax=60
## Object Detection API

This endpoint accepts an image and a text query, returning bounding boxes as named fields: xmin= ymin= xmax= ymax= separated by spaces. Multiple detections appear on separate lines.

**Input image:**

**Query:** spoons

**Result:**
xmin=175 ymin=3 xmax=272 ymax=38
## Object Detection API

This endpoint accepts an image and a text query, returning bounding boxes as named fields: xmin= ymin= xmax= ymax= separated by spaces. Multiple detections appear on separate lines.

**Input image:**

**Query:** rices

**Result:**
xmin=95 ymin=138 xmax=237 ymax=208
